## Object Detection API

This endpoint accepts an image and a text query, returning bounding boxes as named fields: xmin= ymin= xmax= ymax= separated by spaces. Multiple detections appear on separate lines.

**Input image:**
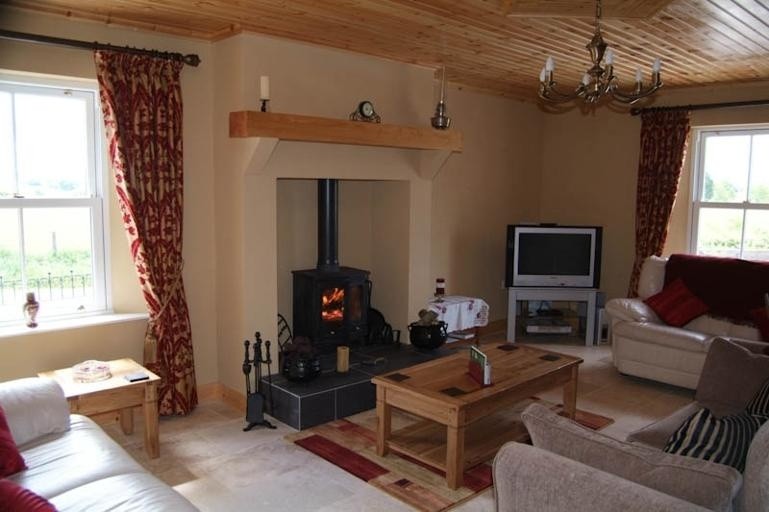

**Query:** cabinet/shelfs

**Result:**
xmin=258 ymin=345 xmax=460 ymax=433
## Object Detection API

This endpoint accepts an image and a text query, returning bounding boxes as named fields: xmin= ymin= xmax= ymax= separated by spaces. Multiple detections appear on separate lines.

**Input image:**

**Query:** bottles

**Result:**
xmin=436 ymin=278 xmax=446 ymax=297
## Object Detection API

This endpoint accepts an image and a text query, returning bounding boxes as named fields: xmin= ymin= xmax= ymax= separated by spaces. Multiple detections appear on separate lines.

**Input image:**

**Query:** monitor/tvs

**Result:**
xmin=505 ymin=225 xmax=602 ymax=289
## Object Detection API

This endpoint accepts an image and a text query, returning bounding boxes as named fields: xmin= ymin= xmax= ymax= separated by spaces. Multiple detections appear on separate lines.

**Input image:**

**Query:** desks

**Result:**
xmin=508 ymin=287 xmax=598 ymax=347
xmin=38 ymin=357 xmax=164 ymax=461
xmin=429 ymin=294 xmax=482 ymax=348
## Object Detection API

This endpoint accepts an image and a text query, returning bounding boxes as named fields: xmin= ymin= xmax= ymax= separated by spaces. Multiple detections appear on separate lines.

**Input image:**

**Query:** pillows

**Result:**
xmin=644 ymin=278 xmax=709 ymax=327
xmin=664 ymin=338 xmax=768 ymax=475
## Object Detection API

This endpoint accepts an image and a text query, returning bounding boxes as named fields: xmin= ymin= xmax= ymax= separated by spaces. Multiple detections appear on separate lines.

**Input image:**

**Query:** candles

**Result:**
xmin=260 ymin=75 xmax=271 ymax=100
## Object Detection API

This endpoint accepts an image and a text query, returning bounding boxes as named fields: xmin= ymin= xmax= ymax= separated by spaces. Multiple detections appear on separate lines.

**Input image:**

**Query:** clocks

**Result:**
xmin=360 ymin=100 xmax=375 ymax=116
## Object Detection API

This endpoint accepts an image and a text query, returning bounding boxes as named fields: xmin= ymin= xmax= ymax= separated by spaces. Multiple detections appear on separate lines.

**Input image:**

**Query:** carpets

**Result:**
xmin=295 ymin=397 xmax=612 ymax=511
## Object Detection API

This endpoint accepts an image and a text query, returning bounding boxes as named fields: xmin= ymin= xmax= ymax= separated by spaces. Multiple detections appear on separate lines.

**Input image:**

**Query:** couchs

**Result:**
xmin=0 ymin=376 xmax=203 ymax=511
xmin=605 ymin=254 xmax=769 ymax=391
xmin=492 ymin=338 xmax=769 ymax=511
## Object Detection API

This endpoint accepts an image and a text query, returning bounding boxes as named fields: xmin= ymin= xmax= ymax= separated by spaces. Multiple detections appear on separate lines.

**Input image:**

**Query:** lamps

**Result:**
xmin=536 ymin=0 xmax=664 ymax=115
